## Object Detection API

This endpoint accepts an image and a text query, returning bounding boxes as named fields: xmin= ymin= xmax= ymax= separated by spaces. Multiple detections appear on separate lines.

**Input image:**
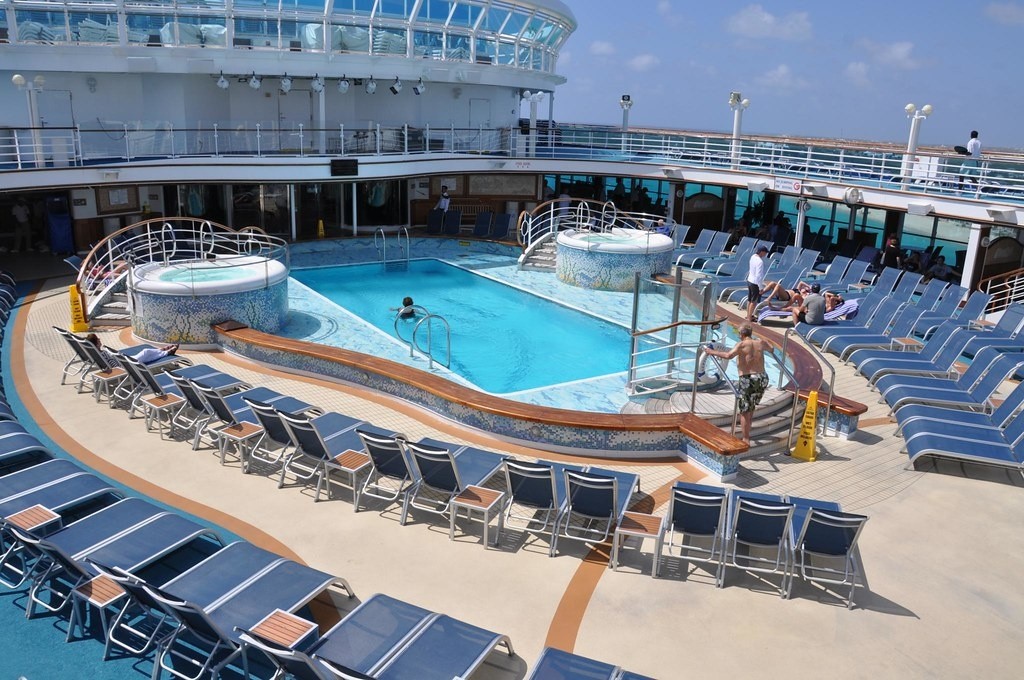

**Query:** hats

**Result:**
xmin=756 ymin=244 xmax=770 ymax=252
xmin=811 ymin=282 xmax=820 ymax=291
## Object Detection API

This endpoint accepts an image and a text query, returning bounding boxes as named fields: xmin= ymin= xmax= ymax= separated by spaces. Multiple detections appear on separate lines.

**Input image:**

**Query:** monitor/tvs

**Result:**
xmin=330 ymin=159 xmax=358 ymax=176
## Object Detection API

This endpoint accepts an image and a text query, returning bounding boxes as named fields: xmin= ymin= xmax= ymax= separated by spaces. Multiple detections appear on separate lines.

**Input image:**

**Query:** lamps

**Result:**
xmin=747 ymin=181 xmax=770 ymax=193
xmin=216 ymin=75 xmax=230 ymax=90
xmin=659 ymin=168 xmax=681 ymax=178
xmin=908 ymin=202 xmax=935 ymax=216
xmin=336 ymin=79 xmax=350 ymax=94
xmin=390 ymin=79 xmax=403 ymax=96
xmin=795 ymin=184 xmax=827 ymax=194
xmin=365 ymin=80 xmax=377 ymax=94
xmin=311 ymin=77 xmax=325 ymax=93
xmin=412 ymin=81 xmax=426 ymax=95
xmin=278 ymin=76 xmax=294 ymax=95
xmin=248 ymin=76 xmax=261 ymax=91
xmin=103 ymin=171 xmax=120 ymax=181
xmin=490 ymin=161 xmax=506 ymax=169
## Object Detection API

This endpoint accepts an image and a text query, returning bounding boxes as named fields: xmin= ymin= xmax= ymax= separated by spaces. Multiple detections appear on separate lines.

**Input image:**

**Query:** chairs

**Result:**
xmin=447 ymin=47 xmax=470 ymax=61
xmin=78 ymin=18 xmax=150 ymax=46
xmin=7 ymin=20 xmax=61 ymax=44
xmin=365 ymin=28 xmax=426 ymax=57
xmin=519 ymin=118 xmax=563 ymax=147
xmin=0 ymin=149 xmax=1024 ymax=680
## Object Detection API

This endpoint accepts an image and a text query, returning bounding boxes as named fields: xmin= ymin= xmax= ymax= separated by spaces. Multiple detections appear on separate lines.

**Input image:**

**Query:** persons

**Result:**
xmin=768 ymin=211 xmax=791 ymax=242
xmin=743 ymin=206 xmax=752 ymax=234
xmin=558 ymin=187 xmax=572 ymax=217
xmin=655 ymin=218 xmax=669 ymax=234
xmin=612 ymin=178 xmax=625 ymax=218
xmin=575 ymin=177 xmax=605 ymax=212
xmin=542 ymin=179 xmax=555 ymax=204
xmin=746 ymin=246 xmax=770 ymax=321
xmin=802 ymin=217 xmax=816 ymax=247
xmin=398 ymin=297 xmax=416 ymax=319
xmin=87 ymin=333 xmax=179 ymax=366
xmin=880 ymin=233 xmax=901 ymax=271
xmin=635 ymin=185 xmax=648 ymax=199
xmin=954 ymin=131 xmax=982 ymax=194
xmin=924 ymin=255 xmax=962 ymax=282
xmin=903 ymin=251 xmax=922 ymax=272
xmin=705 ymin=323 xmax=774 ymax=447
xmin=434 ymin=185 xmax=450 ymax=232
xmin=10 ymin=197 xmax=34 ymax=253
xmin=759 ymin=282 xmax=844 ymax=329
xmin=81 ymin=259 xmax=128 ymax=279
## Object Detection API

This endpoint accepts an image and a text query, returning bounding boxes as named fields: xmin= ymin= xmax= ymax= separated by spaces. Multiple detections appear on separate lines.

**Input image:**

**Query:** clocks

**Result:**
xmin=843 ymin=187 xmax=859 ymax=205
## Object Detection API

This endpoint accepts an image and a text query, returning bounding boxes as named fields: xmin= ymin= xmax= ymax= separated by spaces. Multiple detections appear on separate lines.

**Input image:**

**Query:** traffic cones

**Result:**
xmin=69 ymin=284 xmax=89 ymax=332
xmin=318 ymin=220 xmax=324 ymax=237
xmin=791 ymin=391 xmax=818 ymax=461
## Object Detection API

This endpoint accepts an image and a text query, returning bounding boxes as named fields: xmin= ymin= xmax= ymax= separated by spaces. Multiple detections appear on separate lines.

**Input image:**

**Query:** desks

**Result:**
xmin=0 ymin=504 xmax=63 ymax=580
xmin=71 ymin=574 xmax=127 ymax=649
xmin=982 ymin=397 xmax=1005 ymax=415
xmin=719 ymin=251 xmax=736 ymax=260
xmin=143 ymin=392 xmax=189 ymax=440
xmin=679 ymin=243 xmax=695 ymax=250
xmin=239 ymin=608 xmax=319 ymax=680
xmin=848 ymin=283 xmax=874 ymax=293
xmin=805 ymin=271 xmax=826 ymax=281
xmin=967 ymin=320 xmax=996 ymax=332
xmin=449 ymin=484 xmax=506 ymax=550
xmin=218 ymin=421 xmax=269 ymax=474
xmin=613 ymin=510 xmax=665 ymax=578
xmin=324 ymin=449 xmax=378 ymax=512
xmin=890 ymin=337 xmax=924 ymax=351
xmin=91 ymin=367 xmax=133 ymax=409
xmin=948 ymin=366 xmax=967 ymax=381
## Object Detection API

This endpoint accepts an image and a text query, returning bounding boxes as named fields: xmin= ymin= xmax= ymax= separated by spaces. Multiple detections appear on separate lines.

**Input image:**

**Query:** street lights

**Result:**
xmin=621 ymin=94 xmax=633 ymax=153
xmin=12 ymin=75 xmax=45 ymax=167
xmin=729 ymin=92 xmax=750 ymax=171
xmin=901 ymin=104 xmax=932 ymax=191
xmin=523 ymin=91 xmax=545 ymax=157
